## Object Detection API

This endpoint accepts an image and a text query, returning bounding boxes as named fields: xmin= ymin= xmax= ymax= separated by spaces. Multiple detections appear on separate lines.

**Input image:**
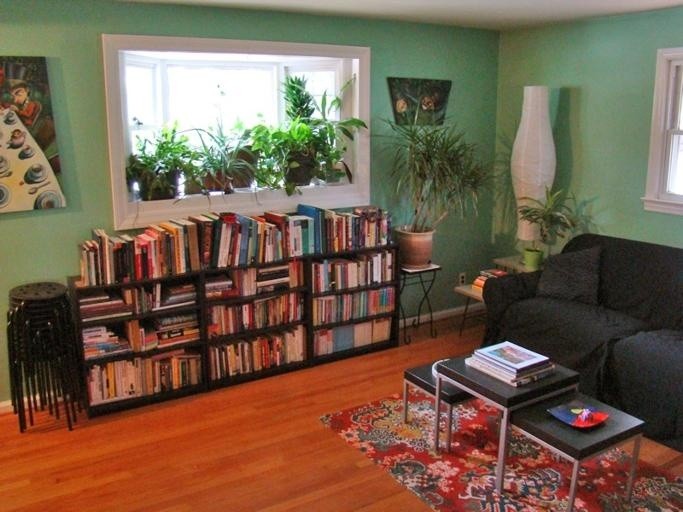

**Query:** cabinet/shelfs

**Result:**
xmin=69 ymin=244 xmax=400 ymax=419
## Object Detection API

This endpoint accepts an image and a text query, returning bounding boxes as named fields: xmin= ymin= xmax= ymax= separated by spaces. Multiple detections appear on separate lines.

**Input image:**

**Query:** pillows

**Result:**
xmin=537 ymin=246 xmax=601 ymax=305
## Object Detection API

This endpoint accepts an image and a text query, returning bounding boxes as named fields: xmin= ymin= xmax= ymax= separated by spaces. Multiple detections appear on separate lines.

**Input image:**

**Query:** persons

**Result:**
xmin=9 ymin=83 xmax=37 ymax=127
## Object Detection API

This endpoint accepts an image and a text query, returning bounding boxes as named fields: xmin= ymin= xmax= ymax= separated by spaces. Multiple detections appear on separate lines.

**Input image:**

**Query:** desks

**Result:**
xmin=399 ymin=263 xmax=441 ymax=345
xmin=453 ymin=285 xmax=486 ymax=337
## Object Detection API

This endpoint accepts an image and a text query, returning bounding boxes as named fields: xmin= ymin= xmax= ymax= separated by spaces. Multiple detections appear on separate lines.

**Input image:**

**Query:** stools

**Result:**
xmin=6 ymin=283 xmax=82 ymax=433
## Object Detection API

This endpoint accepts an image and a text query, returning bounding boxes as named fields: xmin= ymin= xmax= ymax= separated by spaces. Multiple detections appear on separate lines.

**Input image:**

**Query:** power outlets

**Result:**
xmin=459 ymin=273 xmax=466 ymax=285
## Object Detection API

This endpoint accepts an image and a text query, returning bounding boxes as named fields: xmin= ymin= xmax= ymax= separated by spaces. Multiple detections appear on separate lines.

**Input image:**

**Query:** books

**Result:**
xmin=79 ymin=205 xmax=396 ymax=285
xmin=206 ymin=260 xmax=306 ymax=380
xmin=79 ymin=283 xmax=201 ymax=405
xmin=464 ymin=341 xmax=556 ymax=387
xmin=312 ymin=252 xmax=395 ymax=356
xmin=472 ymin=268 xmax=508 ymax=291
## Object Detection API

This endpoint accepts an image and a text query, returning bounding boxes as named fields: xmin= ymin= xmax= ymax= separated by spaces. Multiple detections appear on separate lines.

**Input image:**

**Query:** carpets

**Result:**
xmin=319 ymin=388 xmax=683 ymax=512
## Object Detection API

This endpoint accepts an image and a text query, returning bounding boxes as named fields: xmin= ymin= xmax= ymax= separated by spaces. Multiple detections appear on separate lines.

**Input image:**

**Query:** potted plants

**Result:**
xmin=365 ymin=94 xmax=504 ymax=269
xmin=127 ymin=73 xmax=369 ymax=206
xmin=513 ymin=184 xmax=579 ymax=272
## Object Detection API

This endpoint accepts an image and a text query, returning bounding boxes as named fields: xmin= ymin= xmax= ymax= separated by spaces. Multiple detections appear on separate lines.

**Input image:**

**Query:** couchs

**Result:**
xmin=482 ymin=234 xmax=683 ymax=455
